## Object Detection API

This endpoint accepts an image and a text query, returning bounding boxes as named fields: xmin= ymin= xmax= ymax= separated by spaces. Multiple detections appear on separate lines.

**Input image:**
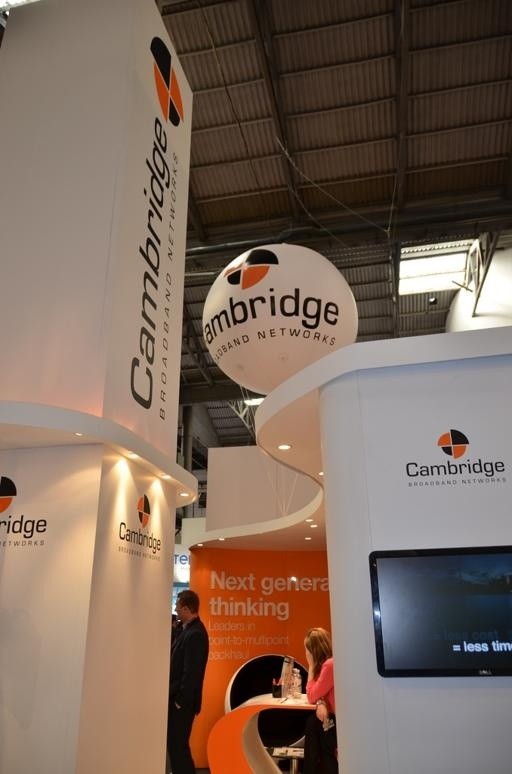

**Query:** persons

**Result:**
xmin=301 ymin=626 xmax=338 ymax=771
xmin=171 ymin=613 xmax=179 ymax=639
xmin=169 ymin=588 xmax=209 ymax=772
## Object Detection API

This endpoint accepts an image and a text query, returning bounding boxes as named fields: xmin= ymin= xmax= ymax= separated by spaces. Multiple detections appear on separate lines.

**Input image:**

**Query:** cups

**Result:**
xmin=272 ymin=684 xmax=282 ymax=698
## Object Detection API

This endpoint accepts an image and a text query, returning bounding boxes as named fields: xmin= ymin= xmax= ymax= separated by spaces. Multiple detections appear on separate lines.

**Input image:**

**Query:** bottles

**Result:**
xmin=289 ymin=668 xmax=303 ymax=698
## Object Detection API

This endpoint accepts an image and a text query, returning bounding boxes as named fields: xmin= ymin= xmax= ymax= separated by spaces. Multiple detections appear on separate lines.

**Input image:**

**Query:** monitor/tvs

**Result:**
xmin=369 ymin=545 xmax=512 ymax=677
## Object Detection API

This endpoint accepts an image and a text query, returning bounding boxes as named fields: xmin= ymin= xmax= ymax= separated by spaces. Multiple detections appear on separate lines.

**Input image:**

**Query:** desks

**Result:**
xmin=207 ymin=694 xmax=316 ymax=774
